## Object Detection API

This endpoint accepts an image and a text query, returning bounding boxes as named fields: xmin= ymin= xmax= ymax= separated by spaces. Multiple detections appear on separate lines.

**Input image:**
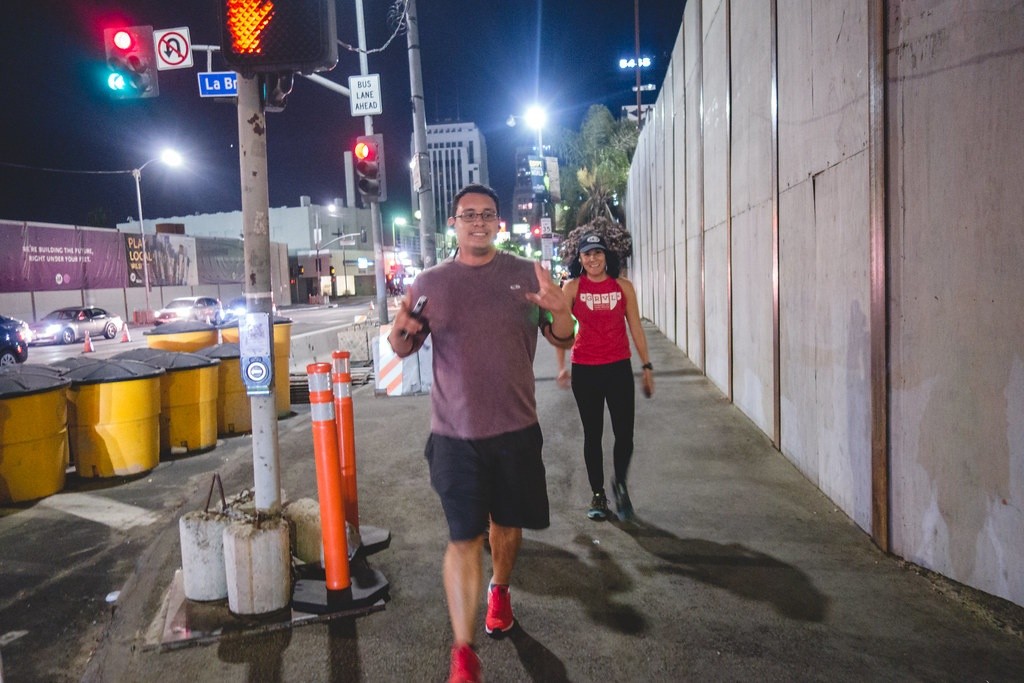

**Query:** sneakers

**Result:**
xmin=447 ymin=642 xmax=485 ymax=683
xmin=485 ymin=584 xmax=515 ymax=635
xmin=613 ymin=478 xmax=633 ymax=520
xmin=588 ymin=494 xmax=611 ymax=519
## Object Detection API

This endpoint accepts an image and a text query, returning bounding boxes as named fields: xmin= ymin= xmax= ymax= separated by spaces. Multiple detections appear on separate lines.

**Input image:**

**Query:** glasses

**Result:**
xmin=454 ymin=211 xmax=499 ymax=223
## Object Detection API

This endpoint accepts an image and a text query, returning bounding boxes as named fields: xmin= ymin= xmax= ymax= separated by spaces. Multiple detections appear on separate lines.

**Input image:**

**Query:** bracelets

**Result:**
xmin=551 ymin=325 xmax=574 ymax=340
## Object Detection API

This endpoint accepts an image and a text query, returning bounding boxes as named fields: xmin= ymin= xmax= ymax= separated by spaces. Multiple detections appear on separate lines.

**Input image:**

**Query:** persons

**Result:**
xmin=386 ymin=185 xmax=575 ymax=683
xmin=557 ymin=235 xmax=653 ymax=521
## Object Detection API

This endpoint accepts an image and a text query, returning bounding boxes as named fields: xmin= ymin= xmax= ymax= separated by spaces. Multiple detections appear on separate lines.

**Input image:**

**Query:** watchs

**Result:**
xmin=642 ymin=362 xmax=652 ymax=370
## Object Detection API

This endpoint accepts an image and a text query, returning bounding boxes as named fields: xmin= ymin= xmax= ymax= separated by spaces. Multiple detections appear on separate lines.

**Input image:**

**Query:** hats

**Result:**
xmin=578 ymin=234 xmax=608 ymax=252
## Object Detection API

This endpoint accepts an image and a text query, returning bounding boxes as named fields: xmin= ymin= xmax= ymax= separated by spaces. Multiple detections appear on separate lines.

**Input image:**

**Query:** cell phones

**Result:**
xmin=402 ymin=295 xmax=427 ymax=339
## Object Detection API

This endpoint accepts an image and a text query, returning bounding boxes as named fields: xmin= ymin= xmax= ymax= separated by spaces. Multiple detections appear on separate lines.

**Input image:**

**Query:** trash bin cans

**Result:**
xmin=65 ymin=360 xmax=164 ymax=478
xmin=0 ymin=371 xmax=72 ymax=504
xmin=146 ymin=352 xmax=221 ymax=455
xmin=4 ymin=348 xmax=170 ymax=371
xmin=200 ymin=344 xmax=252 ymax=434
xmin=223 ymin=314 xmax=292 ymax=419
xmin=144 ymin=320 xmax=219 ymax=352
